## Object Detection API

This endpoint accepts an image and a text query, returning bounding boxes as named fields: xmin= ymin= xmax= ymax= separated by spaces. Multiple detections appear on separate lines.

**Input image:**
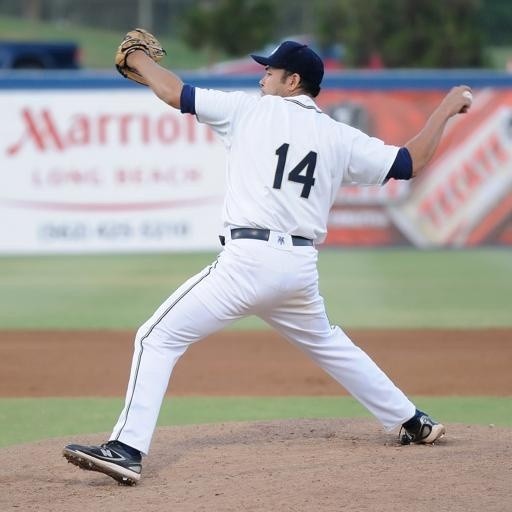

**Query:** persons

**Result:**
xmin=62 ymin=27 xmax=473 ymax=489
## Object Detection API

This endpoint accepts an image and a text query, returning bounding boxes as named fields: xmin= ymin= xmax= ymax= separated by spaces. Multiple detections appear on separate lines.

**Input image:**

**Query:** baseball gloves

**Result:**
xmin=115 ymin=27 xmax=164 ymax=85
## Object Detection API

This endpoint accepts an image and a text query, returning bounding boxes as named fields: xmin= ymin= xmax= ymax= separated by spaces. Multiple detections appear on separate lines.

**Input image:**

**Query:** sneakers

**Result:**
xmin=399 ymin=411 xmax=446 ymax=445
xmin=63 ymin=442 xmax=142 ymax=485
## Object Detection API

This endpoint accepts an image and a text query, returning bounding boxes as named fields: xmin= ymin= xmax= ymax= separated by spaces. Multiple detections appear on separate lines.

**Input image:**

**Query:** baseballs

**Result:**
xmin=463 ymin=90 xmax=473 ymax=108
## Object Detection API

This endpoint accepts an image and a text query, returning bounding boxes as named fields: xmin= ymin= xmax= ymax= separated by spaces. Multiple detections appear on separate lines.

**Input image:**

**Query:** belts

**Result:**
xmin=218 ymin=228 xmax=313 ymax=246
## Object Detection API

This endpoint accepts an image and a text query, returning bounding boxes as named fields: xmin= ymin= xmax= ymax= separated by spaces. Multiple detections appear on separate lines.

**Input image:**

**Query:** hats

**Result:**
xmin=250 ymin=41 xmax=323 ymax=85
